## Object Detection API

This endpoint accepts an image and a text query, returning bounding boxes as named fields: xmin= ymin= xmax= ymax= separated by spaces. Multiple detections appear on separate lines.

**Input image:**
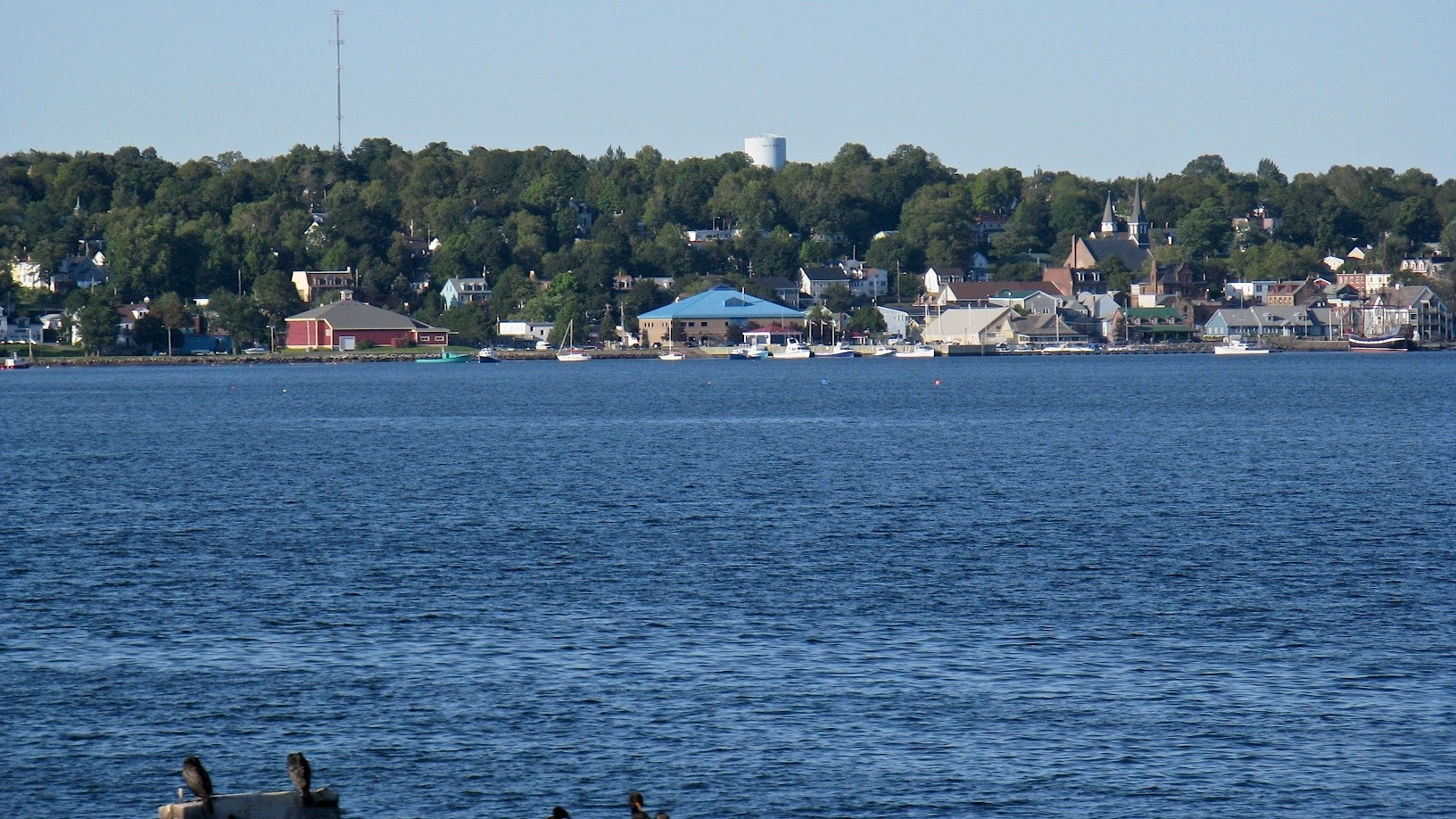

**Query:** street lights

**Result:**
xmin=897 ymin=272 xmax=907 ymax=304
xmin=265 ymin=325 xmax=275 ymax=354
xmin=25 ymin=327 xmax=32 ymax=357
xmin=160 ymin=325 xmax=172 ymax=357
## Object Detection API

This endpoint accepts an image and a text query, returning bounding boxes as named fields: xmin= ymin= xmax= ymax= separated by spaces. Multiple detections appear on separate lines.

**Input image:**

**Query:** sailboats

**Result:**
xmin=658 ymin=320 xmax=684 ymax=360
xmin=1040 ymin=313 xmax=1096 ymax=354
xmin=556 ymin=319 xmax=592 ymax=362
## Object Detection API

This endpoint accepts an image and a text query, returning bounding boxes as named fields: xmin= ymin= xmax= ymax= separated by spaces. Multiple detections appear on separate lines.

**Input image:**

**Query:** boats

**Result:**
xmin=894 ymin=348 xmax=935 ymax=358
xmin=729 ymin=349 xmax=762 ymax=360
xmin=733 ymin=344 xmax=770 ymax=359
xmin=478 ymin=347 xmax=502 ymax=363
xmin=4 ymin=353 xmax=29 ymax=368
xmin=244 ymin=346 xmax=266 ymax=355
xmin=1348 ymin=332 xmax=1410 ymax=351
xmin=772 ymin=337 xmax=811 ymax=359
xmin=1213 ymin=339 xmax=1270 ymax=354
xmin=416 ymin=352 xmax=472 ymax=363
xmin=813 ymin=345 xmax=856 ymax=358
xmin=872 ymin=345 xmax=897 ymax=356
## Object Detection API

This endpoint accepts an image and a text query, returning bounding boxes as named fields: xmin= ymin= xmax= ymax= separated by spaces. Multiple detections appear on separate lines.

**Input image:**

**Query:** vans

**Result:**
xmin=536 ymin=340 xmax=552 ymax=350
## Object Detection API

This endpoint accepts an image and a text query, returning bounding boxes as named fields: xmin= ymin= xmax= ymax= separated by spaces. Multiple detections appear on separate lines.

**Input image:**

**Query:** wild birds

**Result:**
xmin=287 ymin=752 xmax=314 ymax=807
xmin=182 ymin=756 xmax=214 ymax=816
xmin=629 ymin=793 xmax=669 ymax=819
xmin=549 ymin=807 xmax=571 ymax=819
